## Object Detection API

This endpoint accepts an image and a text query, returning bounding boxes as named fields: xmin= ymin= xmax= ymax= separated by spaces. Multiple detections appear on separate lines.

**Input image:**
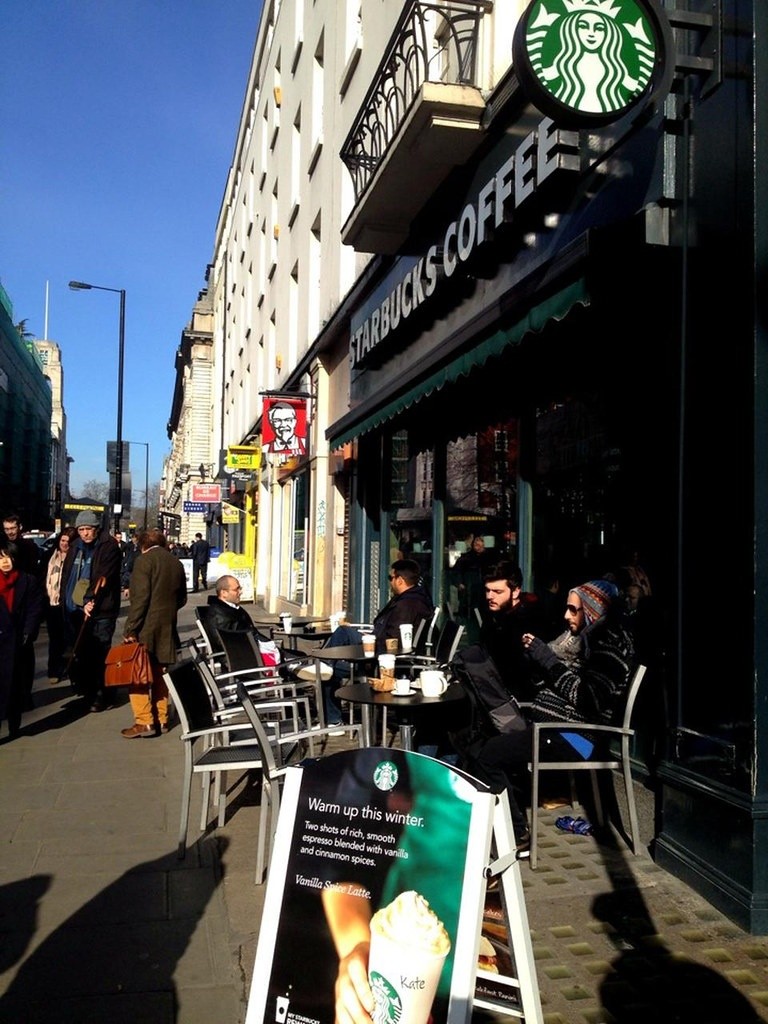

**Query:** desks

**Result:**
xmin=255 ymin=616 xmax=329 ymax=630
xmin=271 ymin=625 xmax=337 ymax=652
xmin=309 ymin=643 xmax=416 ymax=744
xmin=333 ymin=684 xmax=466 ymax=752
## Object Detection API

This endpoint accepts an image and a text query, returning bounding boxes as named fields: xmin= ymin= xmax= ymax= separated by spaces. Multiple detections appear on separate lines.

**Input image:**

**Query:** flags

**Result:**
xmin=221 ymin=502 xmax=239 ymax=524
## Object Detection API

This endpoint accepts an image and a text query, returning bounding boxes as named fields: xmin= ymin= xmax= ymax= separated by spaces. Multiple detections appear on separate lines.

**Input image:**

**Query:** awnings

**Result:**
xmin=329 ymin=274 xmax=593 ymax=453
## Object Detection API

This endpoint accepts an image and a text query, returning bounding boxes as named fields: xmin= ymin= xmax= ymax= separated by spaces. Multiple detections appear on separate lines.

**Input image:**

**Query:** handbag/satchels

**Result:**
xmin=72 ymin=578 xmax=91 ymax=606
xmin=259 ymin=639 xmax=281 ymax=686
xmin=105 ymin=636 xmax=153 ymax=688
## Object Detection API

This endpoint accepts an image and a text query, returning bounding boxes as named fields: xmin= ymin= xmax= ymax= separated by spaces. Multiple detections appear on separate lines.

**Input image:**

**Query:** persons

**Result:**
xmin=115 ymin=533 xmax=189 ymax=593
xmin=205 ymin=576 xmax=307 ymax=680
xmin=402 ymin=526 xmax=517 ymax=614
xmin=0 ymin=507 xmax=121 ymax=740
xmin=313 ymin=749 xmax=475 ymax=1024
xmin=417 ymin=560 xmax=549 ymax=766
xmin=120 ymin=528 xmax=188 ymax=738
xmin=472 ymin=578 xmax=634 ymax=855
xmin=189 ymin=533 xmax=210 ymax=592
xmin=287 ymin=559 xmax=436 ymax=735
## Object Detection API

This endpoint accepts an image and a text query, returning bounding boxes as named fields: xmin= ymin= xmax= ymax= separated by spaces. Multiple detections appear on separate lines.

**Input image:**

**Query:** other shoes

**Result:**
xmin=318 ymin=720 xmax=344 ymax=736
xmin=50 ymin=675 xmax=60 ymax=684
xmin=293 ymin=663 xmax=334 ymax=681
xmin=490 ymin=832 xmax=530 ymax=859
xmin=89 ymin=703 xmax=102 ymax=712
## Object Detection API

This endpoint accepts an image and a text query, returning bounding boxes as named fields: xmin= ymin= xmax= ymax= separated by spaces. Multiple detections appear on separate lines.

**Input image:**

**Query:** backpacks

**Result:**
xmin=452 ymin=646 xmax=528 ymax=735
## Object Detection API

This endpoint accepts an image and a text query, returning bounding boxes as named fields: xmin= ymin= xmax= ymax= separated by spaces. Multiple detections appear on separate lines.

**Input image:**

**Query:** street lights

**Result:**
xmin=68 ymin=279 xmax=126 ymax=534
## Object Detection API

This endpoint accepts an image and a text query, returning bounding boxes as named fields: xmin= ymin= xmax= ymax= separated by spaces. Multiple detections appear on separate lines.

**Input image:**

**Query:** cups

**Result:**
xmin=329 ymin=614 xmax=340 ymax=632
xmin=399 ymin=623 xmax=413 ymax=649
xmin=361 ymin=634 xmax=376 ymax=658
xmin=377 ymin=654 xmax=396 ymax=679
xmin=419 ymin=670 xmax=448 ymax=697
xmin=283 ymin=618 xmax=292 ymax=634
xmin=393 ymin=679 xmax=410 ymax=694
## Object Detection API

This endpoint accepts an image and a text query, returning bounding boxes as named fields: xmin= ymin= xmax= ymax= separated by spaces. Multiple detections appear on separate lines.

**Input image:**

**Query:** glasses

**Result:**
xmin=562 ymin=603 xmax=582 ymax=616
xmin=387 ymin=575 xmax=398 ymax=581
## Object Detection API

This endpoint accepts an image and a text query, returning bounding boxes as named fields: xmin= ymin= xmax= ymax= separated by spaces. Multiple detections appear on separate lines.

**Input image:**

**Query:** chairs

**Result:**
xmin=529 ymin=665 xmax=646 ymax=872
xmin=161 ymin=595 xmax=485 ymax=885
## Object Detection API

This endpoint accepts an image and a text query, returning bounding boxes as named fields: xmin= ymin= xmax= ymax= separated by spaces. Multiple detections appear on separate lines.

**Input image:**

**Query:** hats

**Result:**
xmin=568 ymin=580 xmax=620 ymax=629
xmin=75 ymin=510 xmax=100 ymax=529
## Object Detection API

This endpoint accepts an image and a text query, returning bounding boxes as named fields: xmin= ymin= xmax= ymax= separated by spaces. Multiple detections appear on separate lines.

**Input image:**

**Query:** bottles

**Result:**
xmin=390 ymin=689 xmax=417 ymax=697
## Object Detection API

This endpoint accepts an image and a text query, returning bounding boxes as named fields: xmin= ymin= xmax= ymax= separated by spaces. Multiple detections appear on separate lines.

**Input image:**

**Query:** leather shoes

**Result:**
xmin=121 ymin=724 xmax=155 ymax=739
xmin=158 ymin=722 xmax=169 ymax=734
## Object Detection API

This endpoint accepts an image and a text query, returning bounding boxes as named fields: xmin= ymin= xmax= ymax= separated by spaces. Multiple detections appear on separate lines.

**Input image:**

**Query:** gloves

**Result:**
xmin=23 ymin=633 xmax=36 ymax=647
